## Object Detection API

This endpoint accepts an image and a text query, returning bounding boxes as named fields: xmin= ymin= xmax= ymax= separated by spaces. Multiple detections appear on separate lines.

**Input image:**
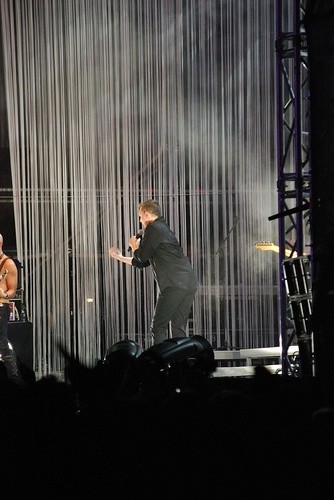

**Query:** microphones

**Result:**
xmin=127 ymin=230 xmax=143 ymax=252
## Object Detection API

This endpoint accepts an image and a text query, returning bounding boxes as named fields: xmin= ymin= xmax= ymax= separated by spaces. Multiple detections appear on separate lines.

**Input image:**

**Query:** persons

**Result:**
xmin=108 ymin=200 xmax=198 ymax=345
xmin=0 ymin=234 xmax=18 ymax=376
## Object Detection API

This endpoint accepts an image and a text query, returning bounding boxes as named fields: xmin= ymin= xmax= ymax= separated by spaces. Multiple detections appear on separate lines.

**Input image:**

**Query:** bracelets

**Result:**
xmin=4 ymin=293 xmax=8 ymax=298
